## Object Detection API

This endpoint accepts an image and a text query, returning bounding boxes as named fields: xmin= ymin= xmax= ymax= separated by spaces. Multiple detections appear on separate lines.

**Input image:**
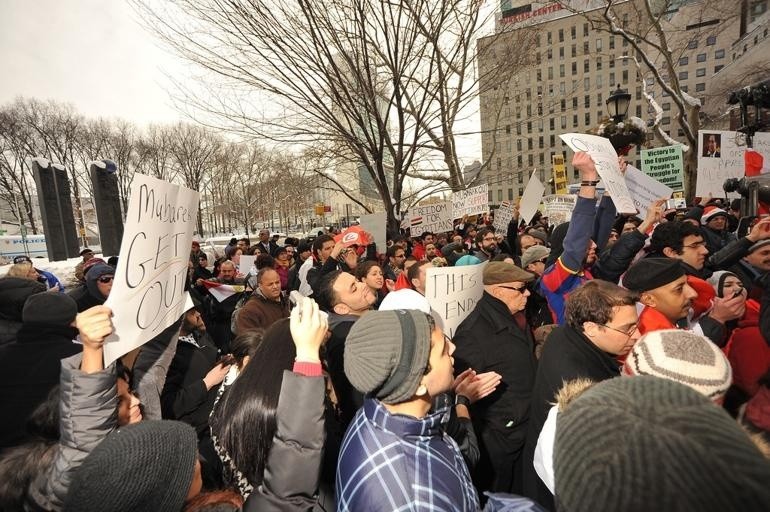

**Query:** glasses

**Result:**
xmin=598 ymin=318 xmax=640 ymax=337
xmin=499 ymin=285 xmax=527 ymax=294
xmin=97 ymin=277 xmax=112 ymax=283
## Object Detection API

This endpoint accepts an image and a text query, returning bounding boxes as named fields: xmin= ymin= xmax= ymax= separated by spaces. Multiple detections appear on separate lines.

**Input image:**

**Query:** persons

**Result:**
xmin=701 ymin=135 xmax=719 ymax=157
xmin=2 ymin=151 xmax=769 ymax=510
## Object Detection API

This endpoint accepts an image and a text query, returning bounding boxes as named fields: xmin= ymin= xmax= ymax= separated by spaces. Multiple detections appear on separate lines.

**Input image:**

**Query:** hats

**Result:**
xmin=23 ymin=292 xmax=78 ymax=325
xmin=62 ymin=419 xmax=198 ymax=512
xmin=343 ymin=309 xmax=432 ymax=406
xmin=621 ymin=329 xmax=733 ymax=401
xmin=622 ymin=205 xmax=738 ymax=295
xmin=483 ymin=244 xmax=553 ymax=285
xmin=554 ymin=376 xmax=768 ymax=510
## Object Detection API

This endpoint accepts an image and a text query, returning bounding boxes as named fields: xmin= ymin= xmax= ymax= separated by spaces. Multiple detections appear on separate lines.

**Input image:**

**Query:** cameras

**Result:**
xmin=666 ymin=198 xmax=686 ymax=210
xmin=288 ymin=290 xmax=305 ymax=313
xmin=341 ymin=248 xmax=348 ymax=254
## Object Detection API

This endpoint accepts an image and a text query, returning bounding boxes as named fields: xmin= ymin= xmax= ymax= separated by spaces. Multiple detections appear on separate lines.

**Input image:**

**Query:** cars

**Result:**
xmin=308 ymin=226 xmax=330 ymax=242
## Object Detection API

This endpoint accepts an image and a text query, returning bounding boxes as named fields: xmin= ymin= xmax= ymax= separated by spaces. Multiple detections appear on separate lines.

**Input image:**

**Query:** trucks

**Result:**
xmin=0 ymin=233 xmax=49 ymax=267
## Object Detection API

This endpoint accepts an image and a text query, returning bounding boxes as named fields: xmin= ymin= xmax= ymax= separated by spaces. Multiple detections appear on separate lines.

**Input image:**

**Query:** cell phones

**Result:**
xmin=55 ymin=282 xmax=59 ymax=286
xmin=733 ymin=287 xmax=743 ymax=297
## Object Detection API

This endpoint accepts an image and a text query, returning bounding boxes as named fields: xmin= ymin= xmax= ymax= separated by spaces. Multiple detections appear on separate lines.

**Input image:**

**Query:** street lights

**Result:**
xmin=605 ymin=84 xmax=632 ymax=155
